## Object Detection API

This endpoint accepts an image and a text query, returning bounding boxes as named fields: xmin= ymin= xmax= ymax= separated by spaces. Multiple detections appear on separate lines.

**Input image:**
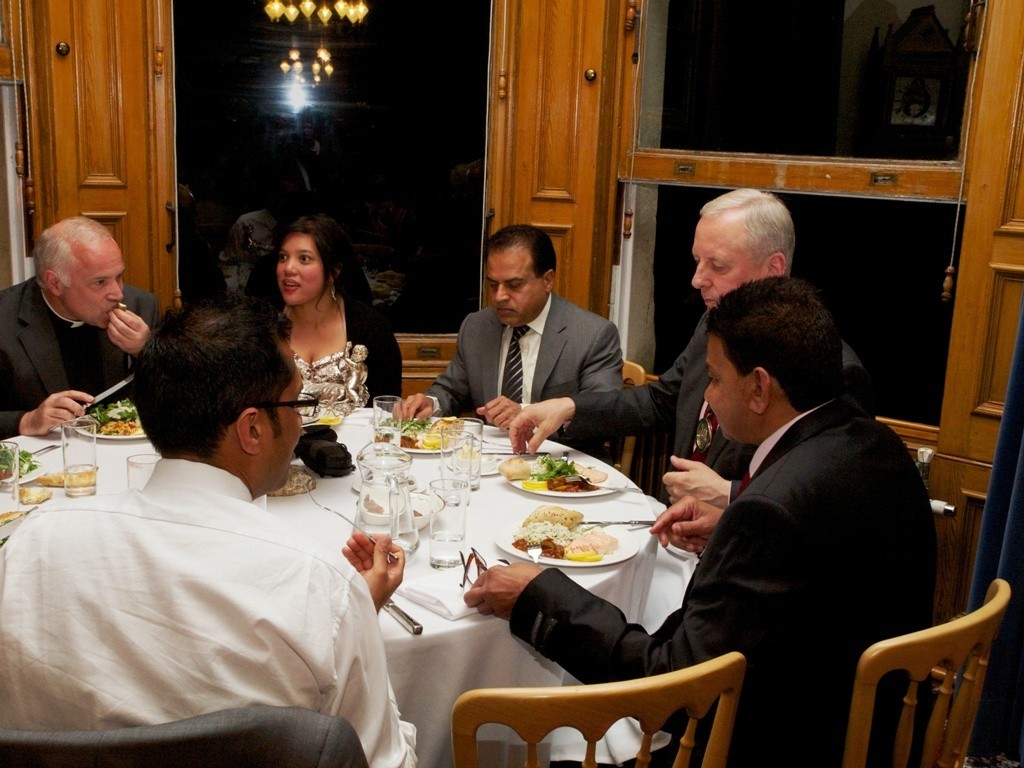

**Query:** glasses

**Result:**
xmin=459 ymin=548 xmax=488 ymax=587
xmin=229 ymin=391 xmax=319 ymax=425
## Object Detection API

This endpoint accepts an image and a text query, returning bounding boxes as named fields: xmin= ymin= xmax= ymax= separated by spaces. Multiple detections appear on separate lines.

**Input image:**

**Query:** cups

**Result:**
xmin=452 ymin=417 xmax=484 ymax=491
xmin=428 ymin=479 xmax=470 ymax=572
xmin=126 ymin=454 xmax=162 ymax=491
xmin=373 ymin=395 xmax=402 ymax=449
xmin=0 ymin=441 xmax=19 ymax=515
xmin=441 ymin=430 xmax=473 ymax=507
xmin=60 ymin=419 xmax=97 ymax=497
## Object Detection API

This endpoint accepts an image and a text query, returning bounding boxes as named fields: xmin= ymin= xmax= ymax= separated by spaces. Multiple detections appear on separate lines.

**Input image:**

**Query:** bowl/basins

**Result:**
xmin=361 ymin=490 xmax=445 ymax=535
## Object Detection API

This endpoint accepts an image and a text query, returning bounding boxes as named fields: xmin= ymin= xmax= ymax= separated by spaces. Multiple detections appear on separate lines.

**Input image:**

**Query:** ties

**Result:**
xmin=738 ymin=470 xmax=751 ymax=496
xmin=501 ymin=326 xmax=532 ymax=403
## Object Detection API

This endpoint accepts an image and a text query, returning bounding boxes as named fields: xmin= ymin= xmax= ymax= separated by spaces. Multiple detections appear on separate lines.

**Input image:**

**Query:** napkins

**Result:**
xmin=394 ymin=558 xmax=509 ymax=621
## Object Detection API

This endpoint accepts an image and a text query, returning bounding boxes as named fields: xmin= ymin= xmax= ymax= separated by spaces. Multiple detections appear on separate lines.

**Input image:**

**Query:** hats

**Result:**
xmin=691 ymin=405 xmax=717 ymax=463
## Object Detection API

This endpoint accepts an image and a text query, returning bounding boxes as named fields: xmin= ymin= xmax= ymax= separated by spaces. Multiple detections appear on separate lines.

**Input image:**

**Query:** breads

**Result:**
xmin=0 ymin=511 xmax=27 ymax=525
xmin=497 ymin=457 xmax=531 ymax=480
xmin=36 ymin=471 xmax=95 ymax=487
xmin=429 ymin=419 xmax=464 ymax=436
xmin=19 ymin=488 xmax=53 ymax=505
xmin=522 ymin=505 xmax=584 ymax=529
xmin=266 ymin=464 xmax=317 ymax=496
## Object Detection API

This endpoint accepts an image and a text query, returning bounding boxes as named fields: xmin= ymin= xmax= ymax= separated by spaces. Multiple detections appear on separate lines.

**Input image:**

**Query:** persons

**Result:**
xmin=178 ymin=110 xmax=484 ymax=350
xmin=0 ymin=217 xmax=159 ymax=441
xmin=509 ymin=187 xmax=873 ymax=512
xmin=393 ymin=225 xmax=623 ymax=468
xmin=269 ymin=215 xmax=402 ymax=412
xmin=0 ymin=299 xmax=418 ymax=768
xmin=464 ymin=276 xmax=936 ymax=768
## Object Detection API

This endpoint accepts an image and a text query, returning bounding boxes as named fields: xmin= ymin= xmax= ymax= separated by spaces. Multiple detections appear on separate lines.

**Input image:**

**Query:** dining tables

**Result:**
xmin=0 ymin=404 xmax=699 ymax=768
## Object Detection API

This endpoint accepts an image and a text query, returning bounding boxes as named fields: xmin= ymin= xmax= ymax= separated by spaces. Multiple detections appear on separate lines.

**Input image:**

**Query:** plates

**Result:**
xmin=75 ymin=415 xmax=147 ymax=440
xmin=299 ymin=408 xmax=344 ymax=426
xmin=446 ymin=449 xmax=519 ymax=475
xmin=493 ymin=516 xmax=640 ymax=567
xmin=19 ymin=455 xmax=49 ymax=485
xmin=504 ymin=459 xmax=628 ymax=497
xmin=377 ymin=420 xmax=468 ymax=454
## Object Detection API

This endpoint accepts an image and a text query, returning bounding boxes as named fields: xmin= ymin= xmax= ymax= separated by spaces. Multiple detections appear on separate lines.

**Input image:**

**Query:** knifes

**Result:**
xmin=481 ymin=452 xmax=551 ymax=455
xmin=560 ymin=451 xmax=569 ymax=462
xmin=578 ymin=520 xmax=656 ymax=525
xmin=80 ymin=373 xmax=134 ymax=408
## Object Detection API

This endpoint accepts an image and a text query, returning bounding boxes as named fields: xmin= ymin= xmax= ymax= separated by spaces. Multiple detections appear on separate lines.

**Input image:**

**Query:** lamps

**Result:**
xmin=265 ymin=0 xmax=369 ymax=31
xmin=280 ymin=34 xmax=333 ymax=81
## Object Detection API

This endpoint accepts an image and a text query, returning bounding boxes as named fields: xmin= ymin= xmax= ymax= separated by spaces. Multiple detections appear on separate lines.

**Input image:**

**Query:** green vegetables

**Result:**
xmin=90 ymin=398 xmax=137 ymax=426
xmin=529 ymin=454 xmax=579 ymax=481
xmin=0 ymin=443 xmax=40 ymax=475
xmin=0 ymin=520 xmax=13 ymax=546
xmin=376 ymin=417 xmax=432 ymax=438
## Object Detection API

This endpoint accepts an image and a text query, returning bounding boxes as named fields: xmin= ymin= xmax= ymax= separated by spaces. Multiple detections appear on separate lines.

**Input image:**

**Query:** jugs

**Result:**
xmin=355 ymin=440 xmax=420 ymax=553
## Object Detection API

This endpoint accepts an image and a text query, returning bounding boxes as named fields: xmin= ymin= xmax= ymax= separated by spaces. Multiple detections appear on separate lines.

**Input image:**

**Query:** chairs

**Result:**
xmin=453 ymin=652 xmax=746 ymax=768
xmin=842 ymin=578 xmax=1011 ymax=768
xmin=0 ymin=708 xmax=367 ymax=768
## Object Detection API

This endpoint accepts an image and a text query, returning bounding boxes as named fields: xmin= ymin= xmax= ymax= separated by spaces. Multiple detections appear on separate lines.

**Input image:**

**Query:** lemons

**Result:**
xmin=523 ymin=481 xmax=547 ymax=490
xmin=565 ymin=551 xmax=602 ymax=562
xmin=422 ymin=435 xmax=448 ymax=449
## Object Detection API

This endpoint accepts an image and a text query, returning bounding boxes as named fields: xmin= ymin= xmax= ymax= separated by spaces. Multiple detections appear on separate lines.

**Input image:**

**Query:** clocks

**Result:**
xmin=851 ymin=2 xmax=968 ymax=160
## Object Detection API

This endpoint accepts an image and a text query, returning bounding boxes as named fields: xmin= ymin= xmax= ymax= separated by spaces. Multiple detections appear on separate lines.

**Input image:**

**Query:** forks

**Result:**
xmin=563 ymin=474 xmax=643 ymax=493
xmin=297 ymin=473 xmax=399 ymax=561
